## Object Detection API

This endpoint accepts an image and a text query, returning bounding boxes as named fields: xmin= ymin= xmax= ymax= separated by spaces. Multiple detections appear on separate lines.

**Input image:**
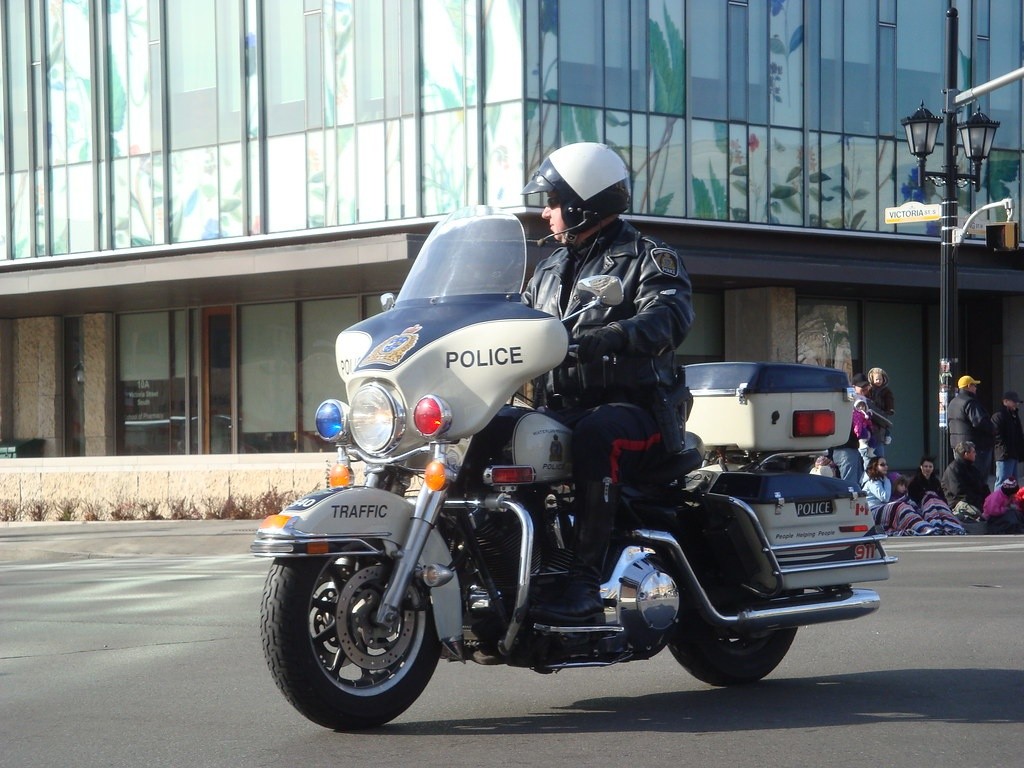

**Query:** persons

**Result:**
xmin=814 ymin=456 xmax=841 ymax=479
xmin=887 ymin=472 xmax=922 ymax=517
xmin=852 ymin=397 xmax=877 ymax=457
xmin=833 ymin=374 xmax=872 ymax=485
xmin=982 ymin=477 xmax=1022 ymax=534
xmin=863 ymin=367 xmax=896 ymax=445
xmin=907 ymin=457 xmax=969 ymax=535
xmin=940 ymin=441 xmax=984 ymax=522
xmin=947 ymin=375 xmax=992 ymax=494
xmin=991 ymin=391 xmax=1024 ymax=491
xmin=521 ymin=142 xmax=695 ymax=628
xmin=858 ymin=456 xmax=932 ymax=535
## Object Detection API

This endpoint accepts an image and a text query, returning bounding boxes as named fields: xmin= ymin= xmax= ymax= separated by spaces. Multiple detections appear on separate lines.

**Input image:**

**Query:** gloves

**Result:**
xmin=571 ymin=320 xmax=625 ymax=365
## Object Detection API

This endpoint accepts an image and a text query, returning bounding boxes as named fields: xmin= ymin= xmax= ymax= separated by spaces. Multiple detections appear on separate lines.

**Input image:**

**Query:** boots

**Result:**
xmin=524 ymin=471 xmax=623 ymax=628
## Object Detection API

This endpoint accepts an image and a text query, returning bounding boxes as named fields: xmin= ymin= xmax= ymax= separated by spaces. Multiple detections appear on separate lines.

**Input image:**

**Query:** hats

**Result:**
xmin=851 ymin=374 xmax=872 ymax=388
xmin=958 ymin=375 xmax=981 ymax=389
xmin=1001 ymin=476 xmax=1018 ymax=494
xmin=1002 ymin=390 xmax=1024 ymax=406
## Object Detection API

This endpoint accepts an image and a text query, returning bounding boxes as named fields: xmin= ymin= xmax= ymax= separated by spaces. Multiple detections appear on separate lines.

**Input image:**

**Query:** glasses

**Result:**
xmin=545 ymin=193 xmax=559 ymax=208
xmin=876 ymin=462 xmax=890 ymax=467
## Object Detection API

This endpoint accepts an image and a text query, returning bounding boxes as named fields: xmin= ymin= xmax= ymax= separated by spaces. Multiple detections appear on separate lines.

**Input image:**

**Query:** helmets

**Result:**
xmin=526 ymin=140 xmax=631 ymax=233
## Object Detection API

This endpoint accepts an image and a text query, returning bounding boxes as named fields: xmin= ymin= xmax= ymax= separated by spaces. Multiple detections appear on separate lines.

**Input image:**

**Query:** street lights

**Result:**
xmin=901 ymin=5 xmax=1001 ymax=505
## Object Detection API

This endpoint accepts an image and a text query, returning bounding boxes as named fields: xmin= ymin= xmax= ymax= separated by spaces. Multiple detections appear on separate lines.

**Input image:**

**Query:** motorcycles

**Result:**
xmin=250 ymin=205 xmax=900 ymax=731
